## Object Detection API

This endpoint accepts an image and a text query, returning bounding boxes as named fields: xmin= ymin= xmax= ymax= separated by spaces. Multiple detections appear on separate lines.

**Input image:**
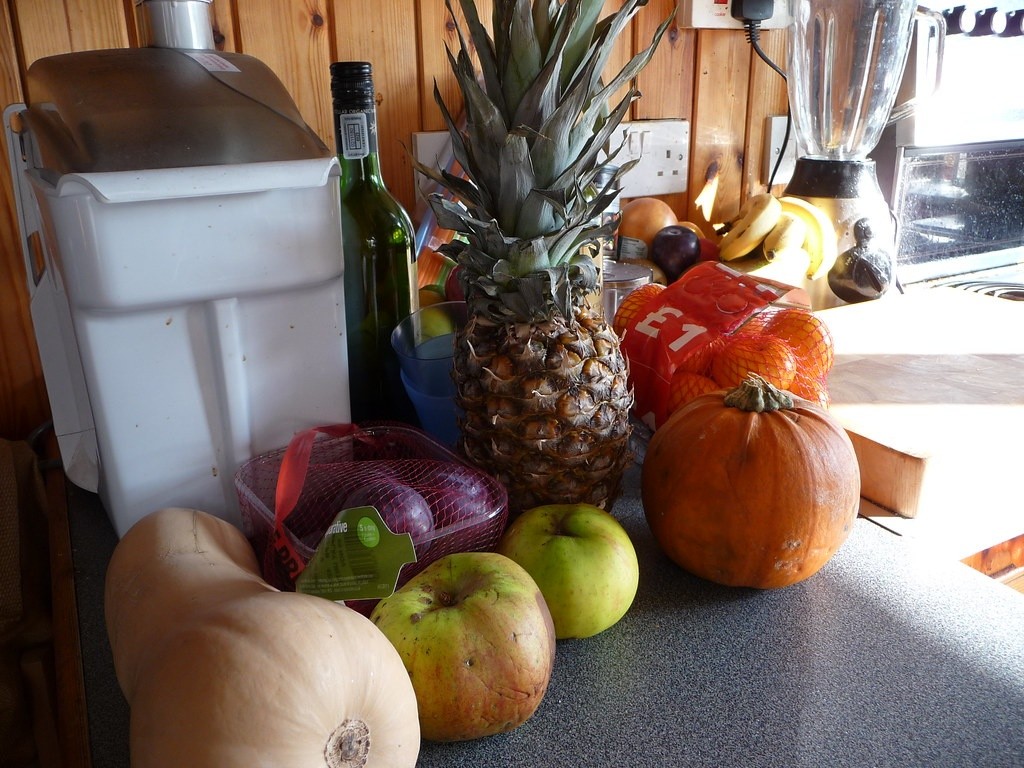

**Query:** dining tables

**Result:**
xmin=43 ymin=377 xmax=1024 ymax=766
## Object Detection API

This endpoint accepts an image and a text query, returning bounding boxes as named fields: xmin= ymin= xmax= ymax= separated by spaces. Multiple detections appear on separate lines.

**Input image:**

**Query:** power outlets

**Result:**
xmin=593 ymin=118 xmax=688 ymax=199
xmin=680 ymin=0 xmax=793 ymax=30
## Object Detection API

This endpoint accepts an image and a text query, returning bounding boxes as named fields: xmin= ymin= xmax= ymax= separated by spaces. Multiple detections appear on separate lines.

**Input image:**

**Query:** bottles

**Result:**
xmin=328 ymin=61 xmax=423 ymax=427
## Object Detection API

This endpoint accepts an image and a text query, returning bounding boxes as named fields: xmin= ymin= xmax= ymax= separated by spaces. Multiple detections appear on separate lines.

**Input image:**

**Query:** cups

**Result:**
xmin=391 ymin=299 xmax=468 ymax=447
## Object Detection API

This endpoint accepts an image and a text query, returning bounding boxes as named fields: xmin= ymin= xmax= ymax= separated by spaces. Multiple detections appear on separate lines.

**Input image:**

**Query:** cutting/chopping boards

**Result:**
xmin=808 ymin=285 xmax=1024 ymax=521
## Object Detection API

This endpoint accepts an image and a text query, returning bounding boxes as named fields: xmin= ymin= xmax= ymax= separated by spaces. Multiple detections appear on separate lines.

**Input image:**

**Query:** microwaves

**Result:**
xmin=872 ymin=136 xmax=1024 ymax=289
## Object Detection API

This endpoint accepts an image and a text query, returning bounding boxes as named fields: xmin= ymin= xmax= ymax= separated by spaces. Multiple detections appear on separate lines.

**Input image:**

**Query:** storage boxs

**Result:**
xmin=20 ymin=45 xmax=353 ymax=556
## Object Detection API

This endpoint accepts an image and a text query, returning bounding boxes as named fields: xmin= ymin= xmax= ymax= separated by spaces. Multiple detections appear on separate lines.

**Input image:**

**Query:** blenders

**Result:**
xmin=778 ymin=0 xmax=946 ymax=277
